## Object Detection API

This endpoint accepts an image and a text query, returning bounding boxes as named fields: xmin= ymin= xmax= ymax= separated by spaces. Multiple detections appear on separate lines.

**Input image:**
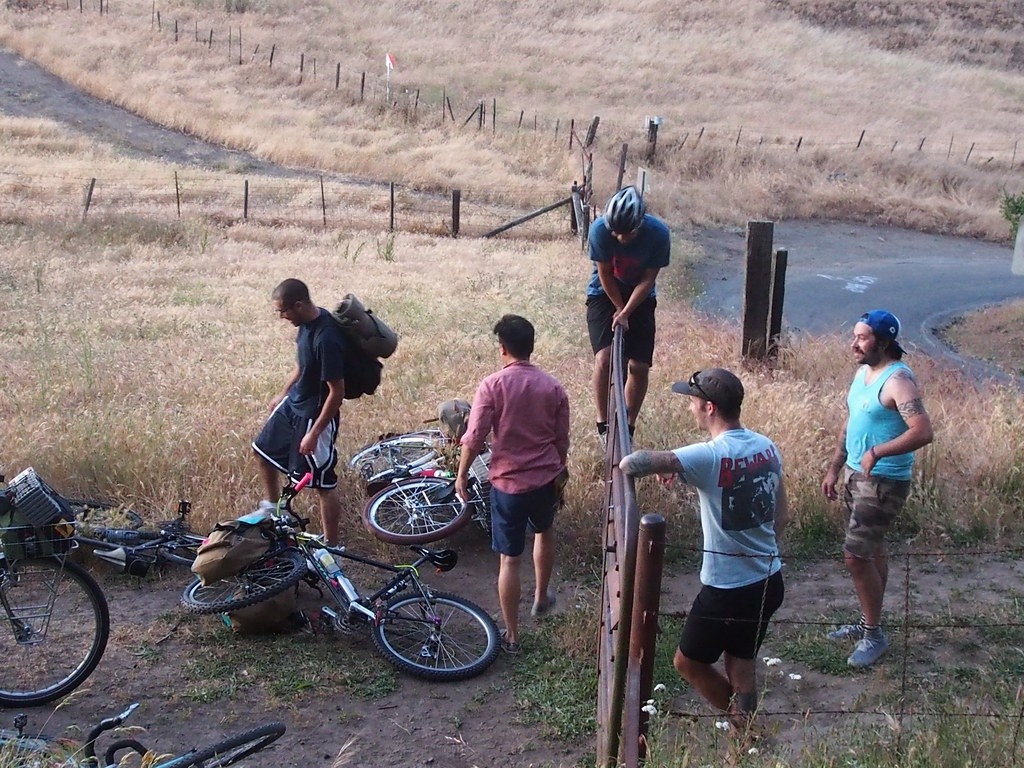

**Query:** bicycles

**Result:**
xmin=182 ymin=458 xmax=503 ymax=682
xmin=0 ymin=465 xmax=110 ymax=708
xmin=81 ymin=702 xmax=287 ymax=768
xmin=348 ymin=403 xmax=496 ymax=547
xmin=67 ymin=497 xmax=210 ymax=579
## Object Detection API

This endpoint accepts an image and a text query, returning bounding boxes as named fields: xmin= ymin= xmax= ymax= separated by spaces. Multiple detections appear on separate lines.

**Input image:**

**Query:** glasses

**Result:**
xmin=689 ymin=370 xmax=715 ymax=404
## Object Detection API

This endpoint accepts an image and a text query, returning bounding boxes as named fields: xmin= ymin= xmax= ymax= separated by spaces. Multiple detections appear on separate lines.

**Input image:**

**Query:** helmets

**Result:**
xmin=602 ymin=185 xmax=645 ymax=234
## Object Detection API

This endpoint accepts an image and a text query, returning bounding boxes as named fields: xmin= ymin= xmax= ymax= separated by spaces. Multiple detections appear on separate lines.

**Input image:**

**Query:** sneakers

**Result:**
xmin=305 ymin=544 xmax=345 ymax=571
xmin=829 ymin=624 xmax=859 ymax=643
xmin=848 ymin=633 xmax=888 ymax=667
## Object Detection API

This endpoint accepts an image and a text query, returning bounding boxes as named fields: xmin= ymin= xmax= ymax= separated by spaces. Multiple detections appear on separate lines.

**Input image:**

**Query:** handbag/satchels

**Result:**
xmin=436 ymin=397 xmax=473 ymax=445
xmin=190 ymin=518 xmax=280 ymax=587
xmin=226 ymin=564 xmax=297 ymax=636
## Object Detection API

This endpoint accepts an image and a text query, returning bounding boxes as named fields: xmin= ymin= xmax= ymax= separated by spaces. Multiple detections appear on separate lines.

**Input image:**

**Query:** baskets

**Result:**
xmin=470 ymin=448 xmax=493 ymax=536
xmin=0 ymin=519 xmax=80 ymax=584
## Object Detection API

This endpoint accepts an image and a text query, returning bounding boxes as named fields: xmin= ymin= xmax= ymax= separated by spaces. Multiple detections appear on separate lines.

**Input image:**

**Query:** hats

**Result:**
xmin=671 ymin=368 xmax=744 ymax=410
xmin=858 ymin=309 xmax=909 ymax=356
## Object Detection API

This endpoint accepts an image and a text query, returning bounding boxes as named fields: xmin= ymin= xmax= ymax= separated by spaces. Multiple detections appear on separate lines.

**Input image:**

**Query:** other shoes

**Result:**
xmin=531 ymin=589 xmax=556 ymax=618
xmin=596 ymin=426 xmax=608 ymax=451
xmin=499 ymin=628 xmax=520 ymax=654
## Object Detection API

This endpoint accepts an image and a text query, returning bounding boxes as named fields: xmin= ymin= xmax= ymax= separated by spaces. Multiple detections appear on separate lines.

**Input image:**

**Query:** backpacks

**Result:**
xmin=306 ymin=305 xmax=384 ymax=400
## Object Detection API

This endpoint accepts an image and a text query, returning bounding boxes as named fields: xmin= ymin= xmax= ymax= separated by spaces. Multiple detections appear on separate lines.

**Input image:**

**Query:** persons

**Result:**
xmin=250 ymin=277 xmax=345 ymax=559
xmin=455 ymin=313 xmax=570 ymax=653
xmin=619 ymin=367 xmax=792 ymax=768
xmin=821 ymin=308 xmax=933 ymax=666
xmin=585 ymin=182 xmax=670 ymax=451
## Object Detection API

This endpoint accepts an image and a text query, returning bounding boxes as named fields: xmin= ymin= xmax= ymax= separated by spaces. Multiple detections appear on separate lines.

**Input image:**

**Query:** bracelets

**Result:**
xmin=869 ymin=445 xmax=881 ymax=461
